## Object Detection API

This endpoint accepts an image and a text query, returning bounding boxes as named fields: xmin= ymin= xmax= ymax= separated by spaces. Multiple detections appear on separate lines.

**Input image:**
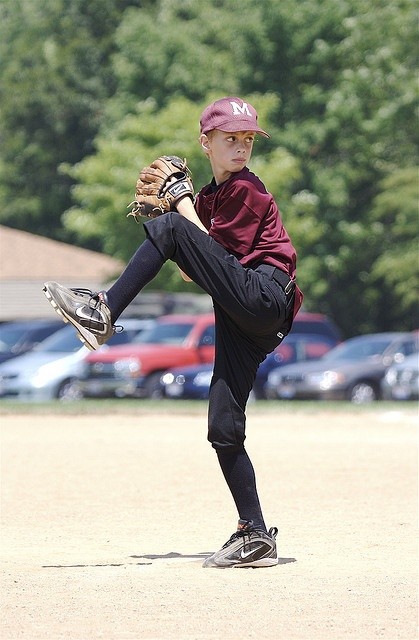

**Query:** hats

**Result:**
xmin=200 ymin=98 xmax=271 ymax=139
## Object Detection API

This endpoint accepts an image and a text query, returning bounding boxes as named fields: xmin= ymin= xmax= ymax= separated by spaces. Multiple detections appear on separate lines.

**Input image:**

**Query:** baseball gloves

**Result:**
xmin=126 ymin=156 xmax=194 ymax=224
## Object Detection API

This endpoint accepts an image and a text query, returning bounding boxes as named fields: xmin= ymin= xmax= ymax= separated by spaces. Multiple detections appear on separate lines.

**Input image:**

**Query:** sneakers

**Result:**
xmin=43 ymin=282 xmax=125 ymax=352
xmin=202 ymin=519 xmax=278 ymax=569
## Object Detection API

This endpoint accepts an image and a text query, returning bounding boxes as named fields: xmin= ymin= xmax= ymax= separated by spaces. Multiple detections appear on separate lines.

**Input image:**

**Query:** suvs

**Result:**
xmin=0 ymin=319 xmax=159 ymax=401
xmin=83 ymin=313 xmax=325 ymax=398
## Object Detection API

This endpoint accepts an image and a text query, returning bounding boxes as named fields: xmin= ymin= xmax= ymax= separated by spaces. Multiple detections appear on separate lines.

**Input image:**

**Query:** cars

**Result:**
xmin=264 ymin=332 xmax=419 ymax=402
xmin=161 ymin=333 xmax=339 ymax=399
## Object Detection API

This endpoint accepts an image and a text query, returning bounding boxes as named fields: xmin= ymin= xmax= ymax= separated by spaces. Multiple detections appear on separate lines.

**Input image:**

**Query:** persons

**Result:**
xmin=40 ymin=99 xmax=303 ymax=566
